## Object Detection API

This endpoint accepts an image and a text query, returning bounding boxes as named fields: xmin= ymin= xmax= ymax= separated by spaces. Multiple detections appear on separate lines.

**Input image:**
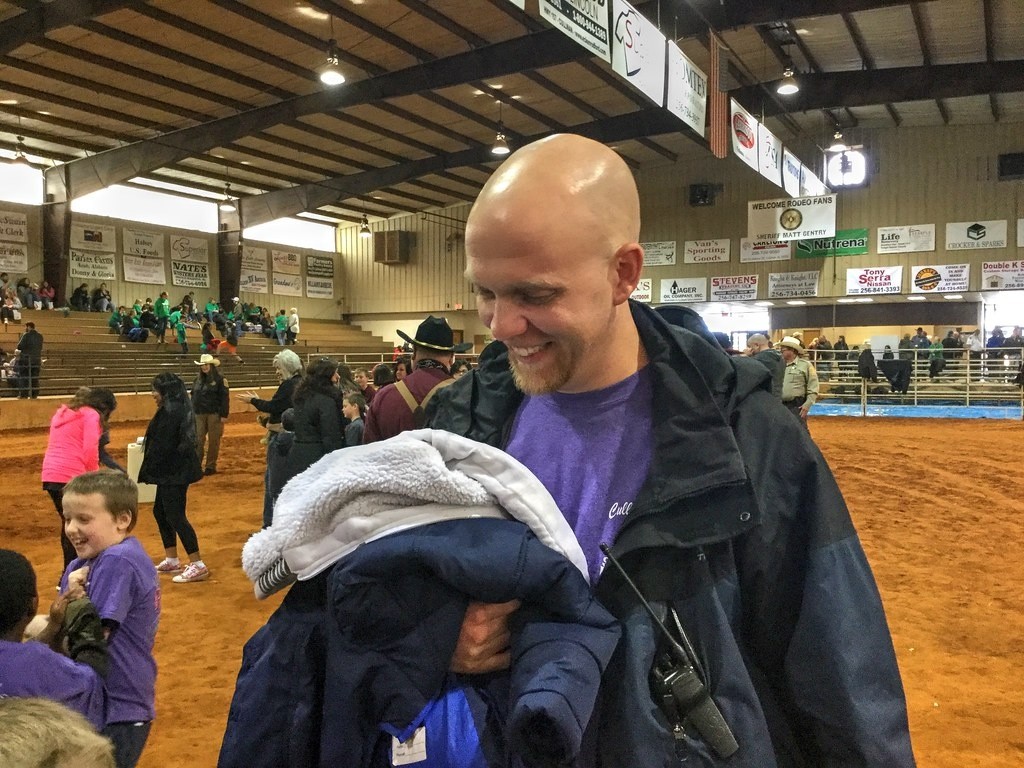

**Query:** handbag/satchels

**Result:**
xmin=128 ymin=327 xmax=148 ymax=342
xmin=12 ymin=308 xmax=22 ymax=320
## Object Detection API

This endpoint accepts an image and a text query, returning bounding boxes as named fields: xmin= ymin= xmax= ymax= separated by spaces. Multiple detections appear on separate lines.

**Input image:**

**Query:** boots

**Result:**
xmin=158 ymin=335 xmax=162 ymax=344
xmin=161 ymin=336 xmax=168 ymax=343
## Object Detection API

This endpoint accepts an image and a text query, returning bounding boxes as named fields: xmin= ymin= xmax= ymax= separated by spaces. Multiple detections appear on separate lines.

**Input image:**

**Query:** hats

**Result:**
xmin=193 ymin=354 xmax=220 ymax=367
xmin=396 ymin=316 xmax=474 ymax=353
xmin=776 ymin=336 xmax=805 ymax=355
xmin=230 ymin=296 xmax=240 ymax=303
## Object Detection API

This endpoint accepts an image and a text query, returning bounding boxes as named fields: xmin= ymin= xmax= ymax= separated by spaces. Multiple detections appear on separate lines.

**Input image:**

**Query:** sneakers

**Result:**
xmin=155 ymin=559 xmax=182 ymax=573
xmin=172 ymin=564 xmax=209 ymax=583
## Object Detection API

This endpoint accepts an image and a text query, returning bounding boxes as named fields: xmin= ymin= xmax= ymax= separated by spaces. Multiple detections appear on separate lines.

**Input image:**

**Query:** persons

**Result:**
xmin=41 ymin=387 xmax=116 ymax=591
xmin=883 ymin=345 xmax=894 ymax=359
xmin=0 ymin=321 xmax=43 ymax=400
xmin=109 ymin=291 xmax=300 ymax=368
xmin=0 ymin=548 xmax=102 ymax=732
xmin=415 ymin=133 xmax=916 ymax=767
xmin=365 ymin=316 xmax=474 ymax=444
xmin=190 ymin=354 xmax=230 ymax=475
xmin=236 ymin=349 xmax=303 ymax=530
xmin=450 ymin=360 xmax=472 ymax=380
xmin=267 ymin=408 xmax=295 ymax=499
xmin=0 ymin=272 xmax=115 ymax=324
xmin=713 ymin=331 xmax=860 ymax=432
xmin=99 ymin=433 xmax=126 ymax=473
xmin=60 ymin=469 xmax=161 ymax=768
xmin=293 ymin=357 xmax=342 ymax=476
xmin=899 ymin=326 xmax=1024 ymax=383
xmin=342 ymin=390 xmax=366 ymax=446
xmin=138 ymin=372 xmax=208 ymax=582
xmin=337 ymin=358 xmax=411 ymax=404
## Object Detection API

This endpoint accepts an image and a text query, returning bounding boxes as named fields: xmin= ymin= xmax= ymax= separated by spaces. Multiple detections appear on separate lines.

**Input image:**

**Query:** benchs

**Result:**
xmin=0 ymin=307 xmax=406 ymax=397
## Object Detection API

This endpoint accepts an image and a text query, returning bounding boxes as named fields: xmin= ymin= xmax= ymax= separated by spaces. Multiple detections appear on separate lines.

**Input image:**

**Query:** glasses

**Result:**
xmin=765 ymin=334 xmax=772 ymax=348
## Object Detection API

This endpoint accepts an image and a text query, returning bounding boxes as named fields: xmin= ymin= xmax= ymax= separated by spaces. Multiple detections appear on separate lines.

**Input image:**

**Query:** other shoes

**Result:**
xmin=205 ymin=468 xmax=216 ymax=476
xmin=4 ymin=318 xmax=9 ymax=325
xmin=31 ymin=393 xmax=37 ymax=398
xmin=241 ymin=361 xmax=248 ymax=365
xmin=17 ymin=393 xmax=28 ymax=399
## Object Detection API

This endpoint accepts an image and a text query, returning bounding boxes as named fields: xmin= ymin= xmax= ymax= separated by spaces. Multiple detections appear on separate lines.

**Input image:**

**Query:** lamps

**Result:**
xmin=218 ymin=165 xmax=237 ymax=212
xmin=13 ymin=115 xmax=30 ymax=167
xmin=320 ymin=14 xmax=346 ymax=86
xmin=776 ymin=44 xmax=799 ymax=95
xmin=491 ymin=99 xmax=510 ymax=155
xmin=832 ymin=131 xmax=847 ymax=152
xmin=359 ymin=200 xmax=371 ymax=236
